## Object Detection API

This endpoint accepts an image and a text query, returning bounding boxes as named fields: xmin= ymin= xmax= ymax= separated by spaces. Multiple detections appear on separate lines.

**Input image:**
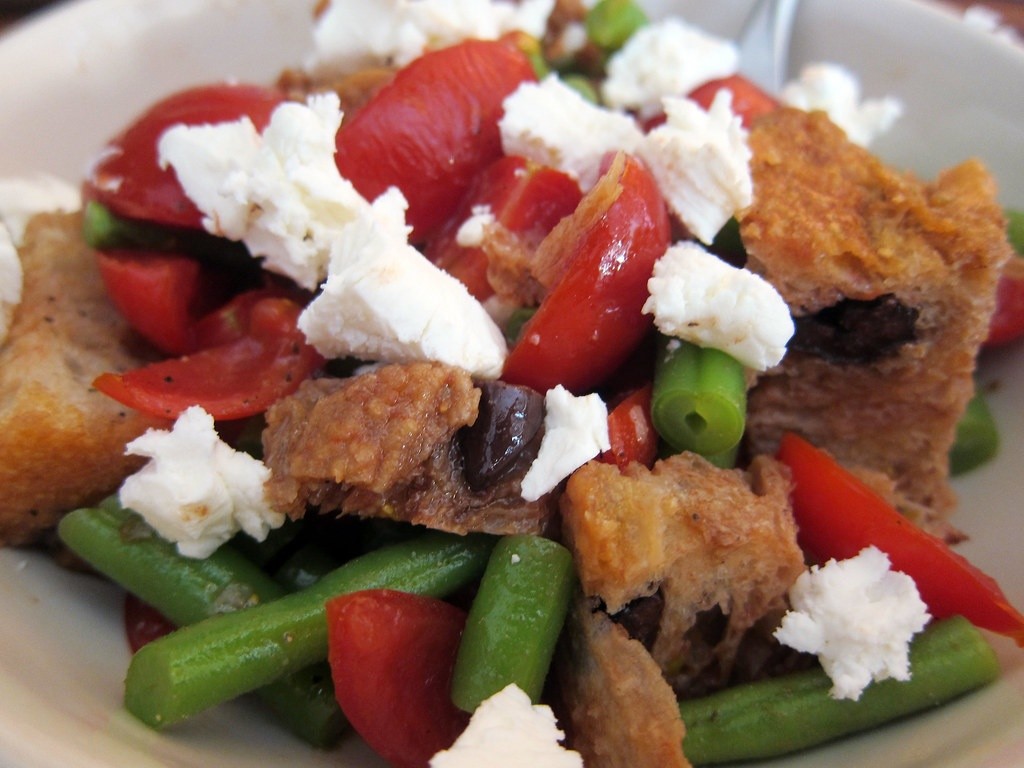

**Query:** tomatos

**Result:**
xmin=82 ymin=37 xmax=1024 ymax=768
